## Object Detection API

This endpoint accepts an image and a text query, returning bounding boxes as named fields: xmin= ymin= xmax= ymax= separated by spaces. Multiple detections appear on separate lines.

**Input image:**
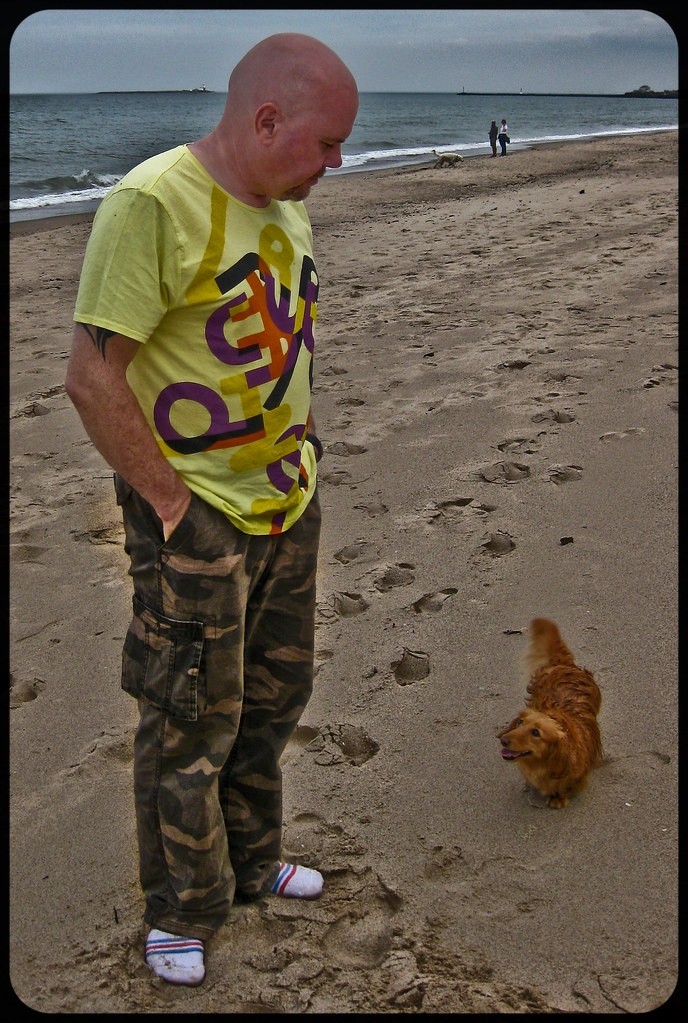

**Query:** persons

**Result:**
xmin=66 ymin=35 xmax=359 ymax=985
xmin=488 ymin=120 xmax=498 ymax=157
xmin=498 ymin=119 xmax=510 ymax=156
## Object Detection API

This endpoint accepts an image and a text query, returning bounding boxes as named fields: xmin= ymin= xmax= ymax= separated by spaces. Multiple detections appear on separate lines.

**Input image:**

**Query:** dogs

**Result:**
xmin=493 ymin=617 xmax=607 ymax=810
xmin=430 ymin=149 xmax=465 ymax=168
